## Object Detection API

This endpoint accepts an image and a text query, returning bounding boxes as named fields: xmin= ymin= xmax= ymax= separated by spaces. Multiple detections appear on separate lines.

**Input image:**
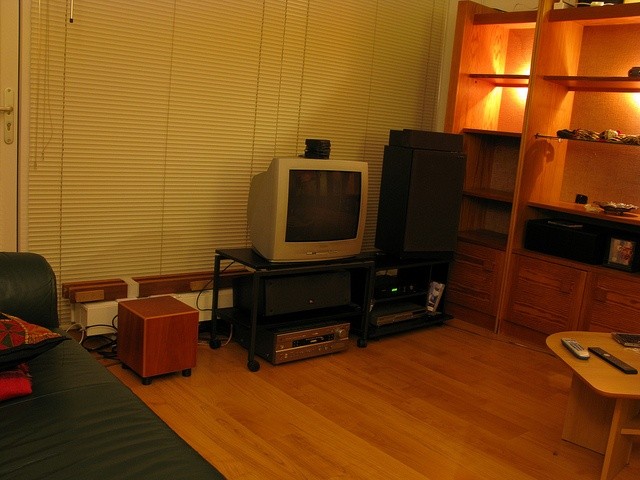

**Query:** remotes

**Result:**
xmin=589 ymin=347 xmax=638 ymax=374
xmin=561 ymin=337 xmax=589 ymax=360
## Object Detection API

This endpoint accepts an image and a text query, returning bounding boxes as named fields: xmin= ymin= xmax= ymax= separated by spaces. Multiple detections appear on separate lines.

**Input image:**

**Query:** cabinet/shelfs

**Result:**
xmin=442 ymin=1 xmax=542 ymax=334
xmin=502 ymin=0 xmax=640 ymax=349
xmin=210 ymin=248 xmax=375 ymax=371
xmin=368 ymin=255 xmax=453 ymax=339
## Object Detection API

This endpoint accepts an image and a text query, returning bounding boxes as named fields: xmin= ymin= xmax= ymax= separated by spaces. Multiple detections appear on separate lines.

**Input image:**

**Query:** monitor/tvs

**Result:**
xmin=247 ymin=157 xmax=369 ymax=263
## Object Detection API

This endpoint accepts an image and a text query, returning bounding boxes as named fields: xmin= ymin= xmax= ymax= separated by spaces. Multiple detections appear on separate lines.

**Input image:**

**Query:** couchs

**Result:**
xmin=2 ymin=252 xmax=229 ymax=476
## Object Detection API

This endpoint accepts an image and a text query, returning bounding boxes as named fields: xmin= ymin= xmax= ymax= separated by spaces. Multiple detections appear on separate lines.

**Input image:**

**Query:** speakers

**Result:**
xmin=389 ymin=128 xmax=464 ymax=152
xmin=372 ymin=145 xmax=467 ymax=253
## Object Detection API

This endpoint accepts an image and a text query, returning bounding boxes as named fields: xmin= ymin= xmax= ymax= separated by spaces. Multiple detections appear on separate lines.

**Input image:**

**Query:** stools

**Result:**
xmin=118 ymin=296 xmax=198 ymax=384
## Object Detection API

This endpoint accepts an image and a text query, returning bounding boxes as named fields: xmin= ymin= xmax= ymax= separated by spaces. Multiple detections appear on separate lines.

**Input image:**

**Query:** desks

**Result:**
xmin=545 ymin=330 xmax=638 ymax=480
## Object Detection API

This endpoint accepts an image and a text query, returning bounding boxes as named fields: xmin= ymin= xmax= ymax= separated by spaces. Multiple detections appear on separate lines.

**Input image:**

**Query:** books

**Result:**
xmin=547 ymin=220 xmax=584 ymax=228
xmin=427 ymin=280 xmax=446 ymax=311
xmin=613 ymin=333 xmax=640 ymax=347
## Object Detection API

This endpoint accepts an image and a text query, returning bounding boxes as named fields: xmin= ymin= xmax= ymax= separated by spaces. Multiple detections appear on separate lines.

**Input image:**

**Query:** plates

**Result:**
xmin=593 ymin=200 xmax=637 ymax=215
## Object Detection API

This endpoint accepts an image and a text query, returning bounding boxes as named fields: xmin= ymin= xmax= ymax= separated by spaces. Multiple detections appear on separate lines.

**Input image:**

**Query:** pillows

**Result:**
xmin=1 ymin=310 xmax=67 ymax=364
